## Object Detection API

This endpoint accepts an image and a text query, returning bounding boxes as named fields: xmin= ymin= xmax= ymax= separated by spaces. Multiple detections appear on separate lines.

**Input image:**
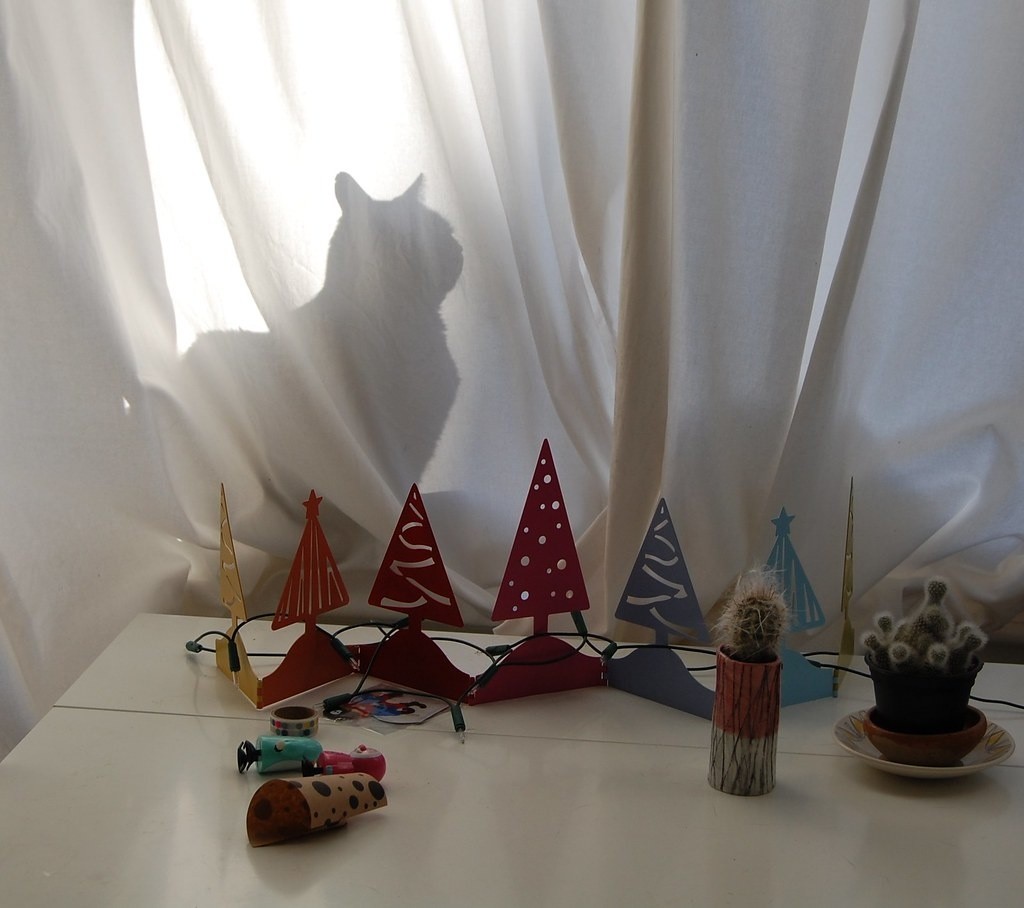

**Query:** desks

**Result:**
xmin=1 ymin=613 xmax=1024 ymax=908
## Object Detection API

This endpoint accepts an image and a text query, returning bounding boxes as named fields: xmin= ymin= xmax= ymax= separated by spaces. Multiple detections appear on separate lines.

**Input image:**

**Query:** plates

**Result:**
xmin=833 ymin=709 xmax=1015 ymax=778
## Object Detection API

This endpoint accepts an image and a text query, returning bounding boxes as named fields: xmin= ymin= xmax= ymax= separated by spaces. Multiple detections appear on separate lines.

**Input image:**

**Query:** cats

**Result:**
xmin=173 ymin=171 xmax=474 ymax=537
